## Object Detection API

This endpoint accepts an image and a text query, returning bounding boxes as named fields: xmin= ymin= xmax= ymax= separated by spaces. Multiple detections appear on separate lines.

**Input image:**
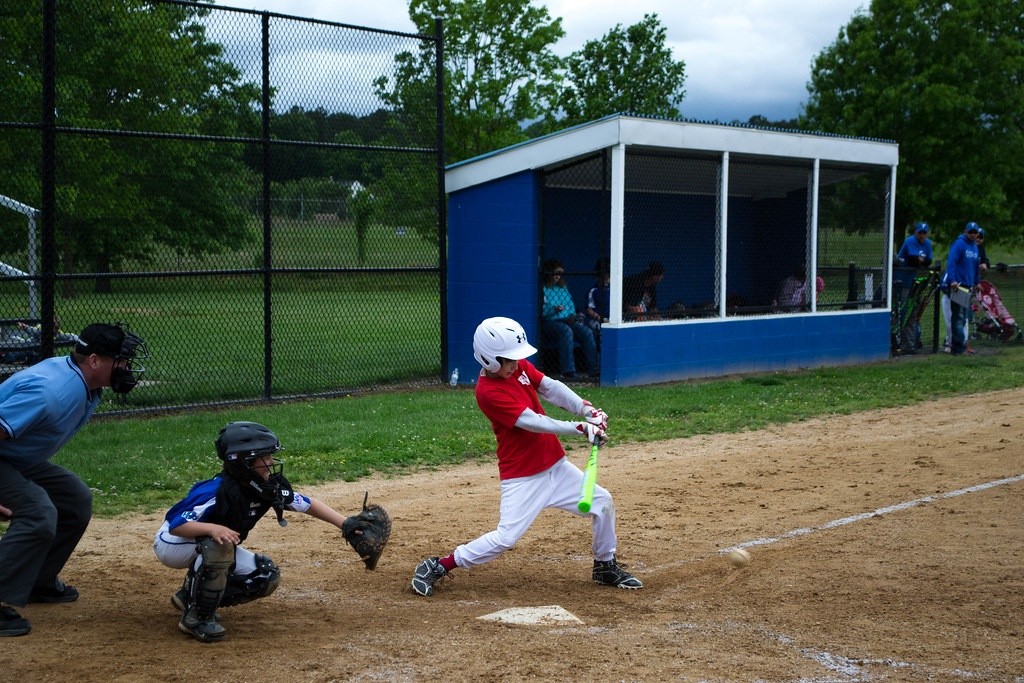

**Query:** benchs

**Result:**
xmin=539 ymin=331 xmax=601 ymax=373
xmin=0 ymin=317 xmax=78 ymax=382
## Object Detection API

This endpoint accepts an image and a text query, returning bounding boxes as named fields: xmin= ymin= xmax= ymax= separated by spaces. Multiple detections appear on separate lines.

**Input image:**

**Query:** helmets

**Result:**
xmin=216 ymin=420 xmax=280 ymax=512
xmin=472 ymin=317 xmax=539 ymax=374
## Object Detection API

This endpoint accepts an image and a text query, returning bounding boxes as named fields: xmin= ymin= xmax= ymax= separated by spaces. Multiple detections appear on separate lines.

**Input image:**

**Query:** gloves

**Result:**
xmin=576 ymin=419 xmax=609 ymax=447
xmin=582 ymin=400 xmax=608 ymax=430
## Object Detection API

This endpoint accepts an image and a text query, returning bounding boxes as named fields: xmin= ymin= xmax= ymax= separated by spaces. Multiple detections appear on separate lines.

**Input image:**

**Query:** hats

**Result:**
xmin=75 ymin=323 xmax=138 ymax=359
xmin=978 ymin=228 xmax=984 ymax=236
xmin=965 ymin=222 xmax=979 ymax=233
xmin=916 ymin=223 xmax=929 ymax=233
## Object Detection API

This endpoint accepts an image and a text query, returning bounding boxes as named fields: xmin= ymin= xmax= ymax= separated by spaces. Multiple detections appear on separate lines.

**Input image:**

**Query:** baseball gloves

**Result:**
xmin=339 ymin=503 xmax=392 ymax=572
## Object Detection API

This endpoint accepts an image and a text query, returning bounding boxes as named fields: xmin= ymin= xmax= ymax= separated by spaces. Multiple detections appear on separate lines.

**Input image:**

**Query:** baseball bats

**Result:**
xmin=577 ymin=434 xmax=600 ymax=513
xmin=891 ymin=271 xmax=943 ymax=341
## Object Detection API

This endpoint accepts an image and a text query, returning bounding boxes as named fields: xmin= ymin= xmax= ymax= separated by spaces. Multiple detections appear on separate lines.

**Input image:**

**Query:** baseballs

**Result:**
xmin=729 ymin=549 xmax=750 ymax=568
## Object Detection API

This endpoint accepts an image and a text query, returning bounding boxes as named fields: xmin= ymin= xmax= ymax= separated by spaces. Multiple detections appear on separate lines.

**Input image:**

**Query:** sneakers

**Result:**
xmin=25 ymin=581 xmax=78 ymax=604
xmin=593 ymin=558 xmax=643 ymax=589
xmin=178 ymin=612 xmax=226 ymax=640
xmin=171 ymin=589 xmax=188 ymax=610
xmin=0 ymin=603 xmax=30 ymax=636
xmin=412 ymin=555 xmax=452 ymax=597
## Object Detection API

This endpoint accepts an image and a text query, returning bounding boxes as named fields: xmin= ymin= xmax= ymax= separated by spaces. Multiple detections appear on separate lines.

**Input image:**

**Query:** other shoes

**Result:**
xmin=966 ymin=347 xmax=977 ymax=354
xmin=564 ymin=371 xmax=575 ymax=378
xmin=956 ymin=352 xmax=971 ymax=357
xmin=944 ymin=346 xmax=952 ymax=353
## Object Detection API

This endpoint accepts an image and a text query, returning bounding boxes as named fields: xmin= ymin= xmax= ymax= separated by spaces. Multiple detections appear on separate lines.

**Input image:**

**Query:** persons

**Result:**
xmin=153 ymin=421 xmax=392 ymax=641
xmin=942 ymin=222 xmax=990 ymax=356
xmin=584 ymin=257 xmax=611 ymax=334
xmin=893 ymin=222 xmax=933 ymax=354
xmin=411 ymin=316 xmax=644 ymax=596
xmin=771 ymin=259 xmax=825 ymax=312
xmin=0 ymin=323 xmax=149 ymax=637
xmin=623 ymin=264 xmax=663 ymax=320
xmin=13 ymin=316 xmax=78 ymax=341
xmin=540 ymin=259 xmax=598 ymax=375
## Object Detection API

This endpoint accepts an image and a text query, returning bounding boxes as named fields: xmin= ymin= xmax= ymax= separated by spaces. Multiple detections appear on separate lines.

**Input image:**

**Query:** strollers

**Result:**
xmin=970 ymin=268 xmax=1024 ymax=345
